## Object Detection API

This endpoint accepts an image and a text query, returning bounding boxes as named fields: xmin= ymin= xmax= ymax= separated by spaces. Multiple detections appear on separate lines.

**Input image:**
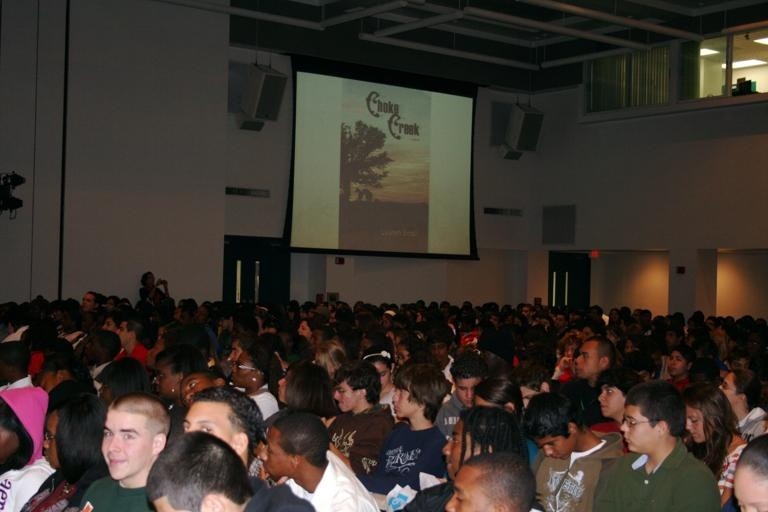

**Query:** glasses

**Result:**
xmin=231 ymin=360 xmax=258 ymax=372
xmin=43 ymin=430 xmax=55 ymax=441
xmin=622 ymin=412 xmax=666 ymax=426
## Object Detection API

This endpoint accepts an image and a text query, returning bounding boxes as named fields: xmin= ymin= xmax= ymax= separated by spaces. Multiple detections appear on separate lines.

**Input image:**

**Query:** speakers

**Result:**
xmin=504 ymin=102 xmax=559 ymax=154
xmin=241 ymin=63 xmax=289 ymax=121
